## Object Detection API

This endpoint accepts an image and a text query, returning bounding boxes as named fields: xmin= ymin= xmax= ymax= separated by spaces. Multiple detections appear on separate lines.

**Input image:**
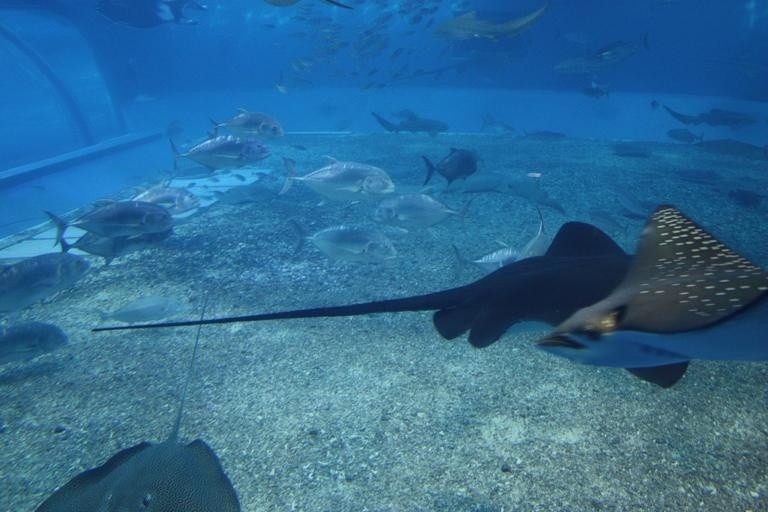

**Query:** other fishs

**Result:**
xmin=0 ymin=0 xmax=768 ymax=391
xmin=34 ymin=272 xmax=240 ymax=512
